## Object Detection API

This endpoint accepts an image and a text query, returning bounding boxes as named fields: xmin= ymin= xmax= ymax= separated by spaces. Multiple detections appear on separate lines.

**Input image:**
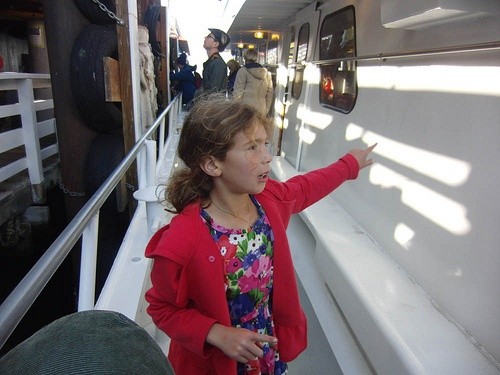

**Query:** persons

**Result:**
xmin=145 ymin=100 xmax=378 ymax=374
xmin=232 ymin=48 xmax=273 ymax=115
xmin=226 ymin=59 xmax=241 ymax=99
xmin=196 ymin=28 xmax=230 ymax=98
xmin=169 ymin=53 xmax=196 ymax=110
xmin=193 ymin=72 xmax=202 ymax=89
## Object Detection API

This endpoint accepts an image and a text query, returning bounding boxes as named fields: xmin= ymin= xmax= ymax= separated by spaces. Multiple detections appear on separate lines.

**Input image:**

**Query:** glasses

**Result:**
xmin=207 ymin=34 xmax=217 ymax=42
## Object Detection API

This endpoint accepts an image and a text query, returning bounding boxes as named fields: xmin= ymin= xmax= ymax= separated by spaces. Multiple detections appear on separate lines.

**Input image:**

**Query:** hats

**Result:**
xmin=177 ymin=53 xmax=186 ymax=65
xmin=208 ymin=28 xmax=230 ymax=47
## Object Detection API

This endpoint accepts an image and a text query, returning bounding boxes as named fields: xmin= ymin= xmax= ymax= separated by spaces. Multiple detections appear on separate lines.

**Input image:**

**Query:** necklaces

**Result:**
xmin=209 ymin=195 xmax=253 ymax=229
